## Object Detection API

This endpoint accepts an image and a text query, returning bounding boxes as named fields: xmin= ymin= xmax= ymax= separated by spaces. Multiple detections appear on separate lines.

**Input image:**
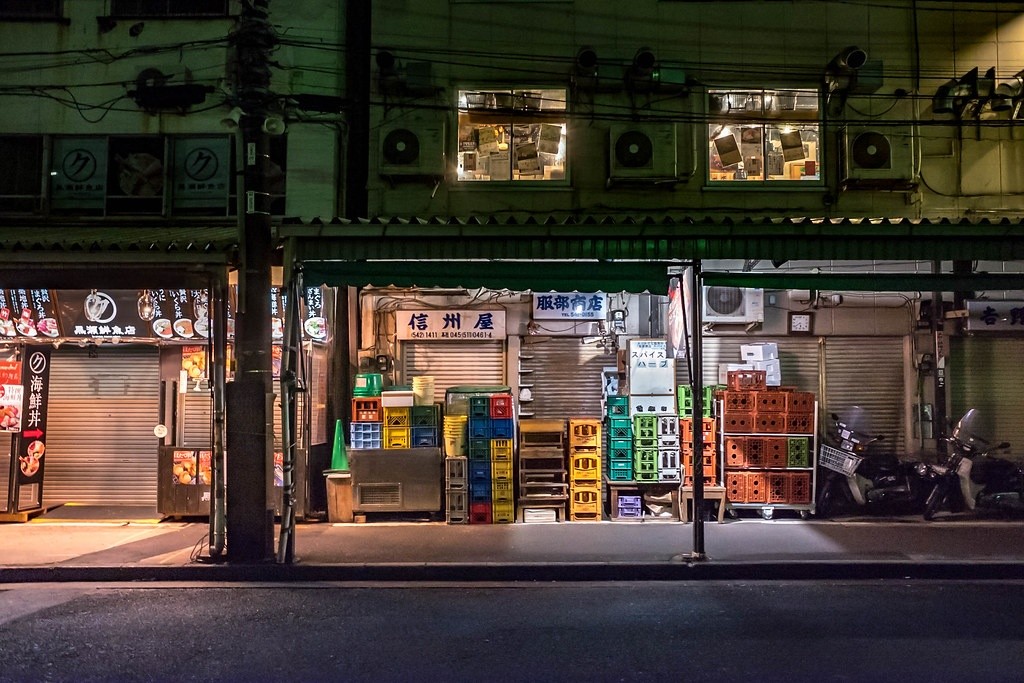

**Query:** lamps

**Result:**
xmin=373 ymin=353 xmax=393 ymax=372
xmin=219 ymin=107 xmax=244 ymax=128
xmin=261 ymin=117 xmax=286 ymax=135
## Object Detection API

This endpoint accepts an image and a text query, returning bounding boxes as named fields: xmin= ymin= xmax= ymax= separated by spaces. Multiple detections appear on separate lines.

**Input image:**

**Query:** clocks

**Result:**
xmin=787 ymin=311 xmax=814 ymax=336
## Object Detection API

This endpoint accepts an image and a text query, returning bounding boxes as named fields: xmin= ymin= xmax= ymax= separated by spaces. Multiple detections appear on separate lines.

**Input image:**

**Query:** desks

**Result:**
xmin=603 ymin=473 xmax=681 ymax=522
xmin=681 ymin=487 xmax=726 ymax=524
xmin=518 ymin=418 xmax=570 ymax=524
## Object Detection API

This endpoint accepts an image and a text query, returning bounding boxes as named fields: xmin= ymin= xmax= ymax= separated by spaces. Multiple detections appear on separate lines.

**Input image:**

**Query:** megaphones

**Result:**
xmin=262 ymin=118 xmax=286 ymax=135
xmin=219 ymin=107 xmax=243 ymax=132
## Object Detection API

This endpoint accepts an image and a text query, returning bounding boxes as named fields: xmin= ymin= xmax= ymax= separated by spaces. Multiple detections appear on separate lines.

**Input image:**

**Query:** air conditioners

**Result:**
xmin=379 ymin=122 xmax=447 ymax=177
xmin=608 ymin=119 xmax=677 ymax=179
xmin=701 ymin=279 xmax=765 ymax=332
xmin=837 ymin=124 xmax=913 ymax=190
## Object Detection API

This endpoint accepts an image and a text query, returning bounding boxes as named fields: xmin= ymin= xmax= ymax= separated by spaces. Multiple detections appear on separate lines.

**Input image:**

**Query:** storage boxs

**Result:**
xmin=350 ymin=384 xmax=440 ymax=450
xmin=712 ymin=342 xmax=814 ymax=504
xmin=567 ymin=417 xmax=603 ymax=520
xmin=600 ymin=336 xmax=716 ymax=517
xmin=442 ymin=382 xmax=515 ymax=524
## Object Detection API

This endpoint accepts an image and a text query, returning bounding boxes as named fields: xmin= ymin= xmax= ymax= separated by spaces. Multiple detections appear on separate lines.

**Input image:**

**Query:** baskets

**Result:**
xmin=819 ymin=444 xmax=866 ymax=478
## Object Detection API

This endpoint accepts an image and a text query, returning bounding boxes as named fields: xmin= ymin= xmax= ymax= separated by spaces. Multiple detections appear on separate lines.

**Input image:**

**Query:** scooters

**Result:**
xmin=816 ymin=404 xmax=935 ymax=520
xmin=913 ymin=408 xmax=1023 ymax=522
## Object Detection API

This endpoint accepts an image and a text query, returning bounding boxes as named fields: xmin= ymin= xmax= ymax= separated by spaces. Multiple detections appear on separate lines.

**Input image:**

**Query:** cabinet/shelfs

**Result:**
xmin=711 ymin=398 xmax=819 ymax=520
xmin=517 ymin=354 xmax=535 ymax=420
